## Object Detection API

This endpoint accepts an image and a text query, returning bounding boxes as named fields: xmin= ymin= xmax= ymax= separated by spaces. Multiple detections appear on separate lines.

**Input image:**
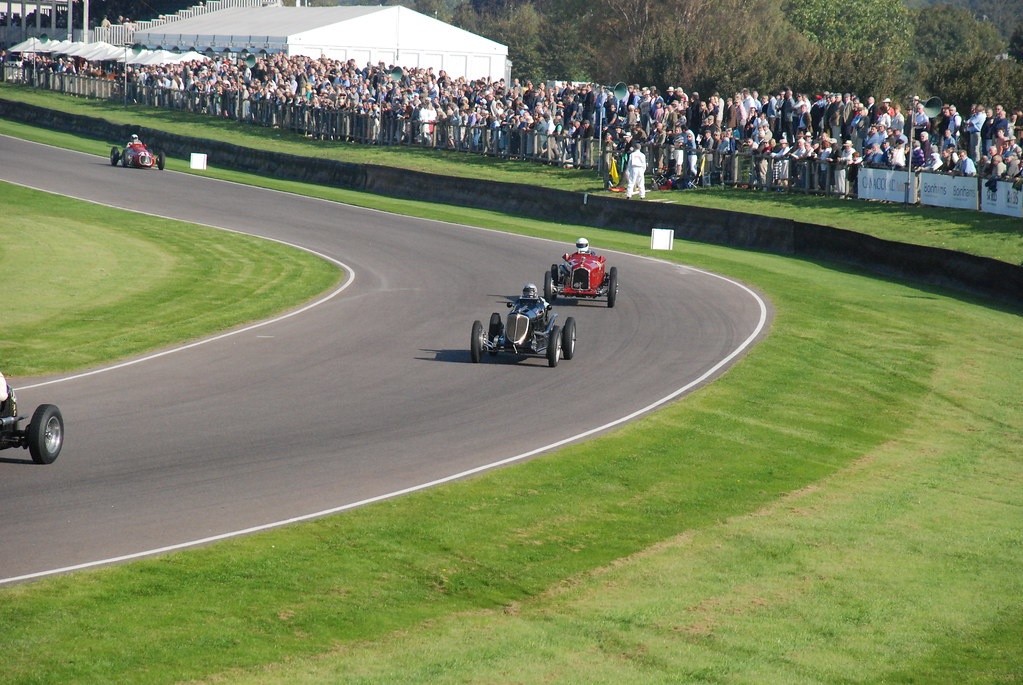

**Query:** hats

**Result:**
xmin=583 ymin=120 xmax=591 ymax=124
xmin=188 ymin=71 xmax=193 ymax=75
xmin=666 ymin=86 xmax=674 ymax=91
xmin=798 ymin=138 xmax=805 ymax=143
xmin=858 ymin=103 xmax=864 ymax=111
xmin=906 ymin=106 xmax=913 ymax=110
xmin=844 ymin=93 xmax=850 ymax=98
xmin=623 ymin=132 xmax=632 ymax=138
xmin=830 ymin=138 xmax=838 ymax=144
xmin=843 ymin=140 xmax=853 ymax=147
xmin=943 ymin=104 xmax=949 ymax=109
xmin=462 ymin=97 xmax=468 ymax=103
xmin=1002 ymin=137 xmax=1009 ymax=142
xmin=671 ymin=100 xmax=678 ymax=104
xmin=883 ymin=98 xmax=890 ymax=103
xmin=675 ymin=87 xmax=683 ymax=92
xmin=805 ymin=132 xmax=812 ymax=137
xmin=628 ymin=85 xmax=634 ymax=88
xmin=779 ymin=139 xmax=787 ymax=144
xmin=1011 ymin=110 xmax=1016 ymax=115
xmin=1011 ymin=135 xmax=1016 ymax=140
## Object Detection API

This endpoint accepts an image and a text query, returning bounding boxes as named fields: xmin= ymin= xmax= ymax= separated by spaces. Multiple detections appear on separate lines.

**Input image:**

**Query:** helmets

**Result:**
xmin=576 ymin=238 xmax=589 ymax=254
xmin=131 ymin=134 xmax=139 ymax=141
xmin=523 ymin=284 xmax=538 ymax=299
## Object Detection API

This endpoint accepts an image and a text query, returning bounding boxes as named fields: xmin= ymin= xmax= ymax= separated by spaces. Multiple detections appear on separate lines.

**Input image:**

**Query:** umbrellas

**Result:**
xmin=8 ymin=38 xmax=211 ymax=66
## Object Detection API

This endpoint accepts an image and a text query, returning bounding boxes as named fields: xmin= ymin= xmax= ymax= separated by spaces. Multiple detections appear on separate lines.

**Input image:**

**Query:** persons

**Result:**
xmin=127 ymin=134 xmax=142 ymax=147
xmin=576 ymin=238 xmax=596 ymax=255
xmin=0 ymin=372 xmax=8 ymax=413
xmin=0 ymin=0 xmax=204 ymax=43
xmin=0 ymin=51 xmax=1023 ymax=199
xmin=523 ymin=284 xmax=549 ymax=308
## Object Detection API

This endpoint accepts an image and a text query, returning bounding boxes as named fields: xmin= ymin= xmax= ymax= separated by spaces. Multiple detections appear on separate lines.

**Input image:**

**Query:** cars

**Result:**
xmin=0 ymin=391 xmax=64 ymax=464
xmin=545 ymin=252 xmax=618 ymax=306
xmin=470 ymin=298 xmax=577 ymax=368
xmin=110 ymin=142 xmax=167 ymax=170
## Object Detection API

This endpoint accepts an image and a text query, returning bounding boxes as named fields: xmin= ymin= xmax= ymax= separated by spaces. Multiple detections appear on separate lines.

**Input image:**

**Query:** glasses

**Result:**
xmin=686 ymin=135 xmax=691 ymax=138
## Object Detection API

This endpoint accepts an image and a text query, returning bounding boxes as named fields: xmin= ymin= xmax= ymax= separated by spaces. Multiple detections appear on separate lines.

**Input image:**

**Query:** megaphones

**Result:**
xmin=242 ymin=54 xmax=256 ymax=68
xmin=605 ymin=82 xmax=627 ymax=100
xmin=917 ymin=97 xmax=943 ymax=119
xmin=383 ymin=66 xmax=403 ymax=81
xmin=35 ymin=33 xmax=48 ymax=43
xmin=129 ymin=43 xmax=142 ymax=55
